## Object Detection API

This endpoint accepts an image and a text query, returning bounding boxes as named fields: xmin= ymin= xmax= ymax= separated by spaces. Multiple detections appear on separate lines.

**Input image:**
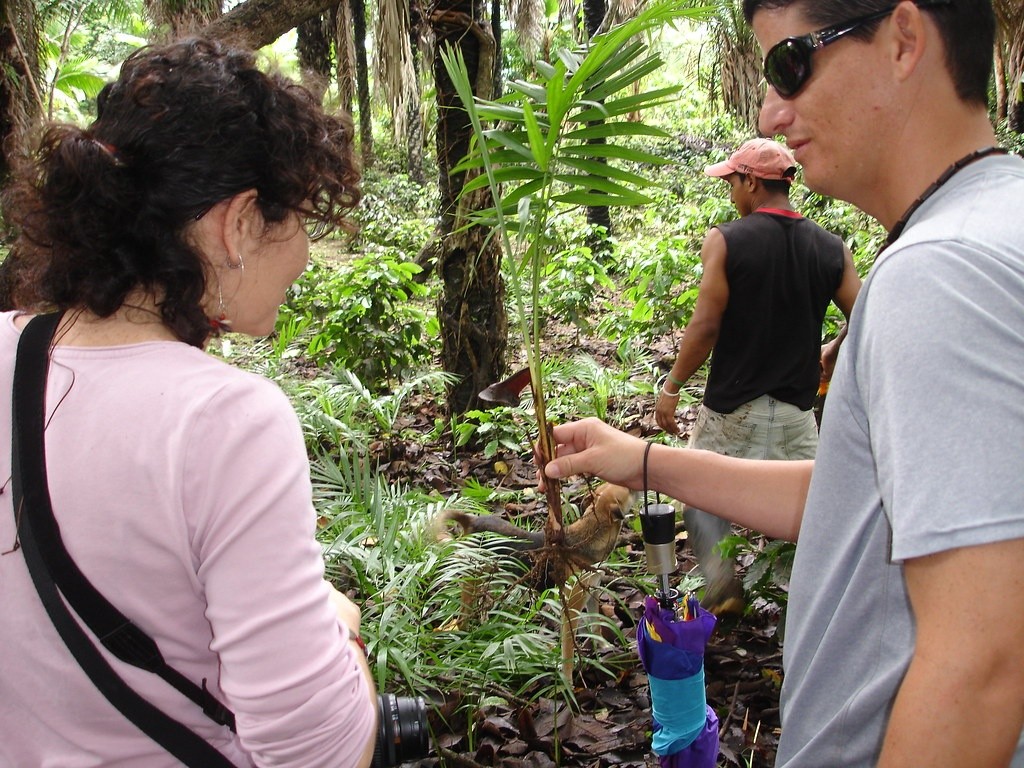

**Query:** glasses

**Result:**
xmin=762 ymin=2 xmax=926 ymax=100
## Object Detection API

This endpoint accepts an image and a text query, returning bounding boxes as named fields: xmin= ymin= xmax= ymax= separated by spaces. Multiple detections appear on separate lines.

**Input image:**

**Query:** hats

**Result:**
xmin=704 ymin=137 xmax=797 ymax=187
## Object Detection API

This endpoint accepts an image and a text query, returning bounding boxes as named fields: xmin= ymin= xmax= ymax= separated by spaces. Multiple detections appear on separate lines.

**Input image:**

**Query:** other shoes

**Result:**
xmin=710 ymin=596 xmax=743 ymax=618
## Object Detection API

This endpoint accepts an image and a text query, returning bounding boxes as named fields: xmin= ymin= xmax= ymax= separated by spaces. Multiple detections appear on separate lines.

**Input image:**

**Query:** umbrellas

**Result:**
xmin=637 ymin=441 xmax=719 ymax=768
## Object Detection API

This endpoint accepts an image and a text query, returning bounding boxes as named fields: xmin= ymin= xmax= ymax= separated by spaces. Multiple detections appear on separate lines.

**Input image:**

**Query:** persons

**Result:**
xmin=534 ymin=0 xmax=1024 ymax=767
xmin=1 ymin=37 xmax=381 ymax=768
xmin=655 ymin=138 xmax=862 ymax=629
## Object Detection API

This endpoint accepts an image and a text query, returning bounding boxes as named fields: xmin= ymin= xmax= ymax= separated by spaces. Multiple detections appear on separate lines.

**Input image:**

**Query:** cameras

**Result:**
xmin=370 ymin=693 xmax=429 ymax=768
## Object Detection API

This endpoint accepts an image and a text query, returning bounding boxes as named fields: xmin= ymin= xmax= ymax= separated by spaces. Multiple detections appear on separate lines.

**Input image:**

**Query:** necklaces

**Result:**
xmin=873 ymin=147 xmax=1009 ymax=262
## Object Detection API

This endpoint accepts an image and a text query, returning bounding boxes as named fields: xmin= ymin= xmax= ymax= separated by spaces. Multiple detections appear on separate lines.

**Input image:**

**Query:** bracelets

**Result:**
xmin=668 ymin=370 xmax=684 ymax=386
xmin=663 ymin=381 xmax=679 ymax=396
xmin=348 ymin=630 xmax=368 ymax=658
